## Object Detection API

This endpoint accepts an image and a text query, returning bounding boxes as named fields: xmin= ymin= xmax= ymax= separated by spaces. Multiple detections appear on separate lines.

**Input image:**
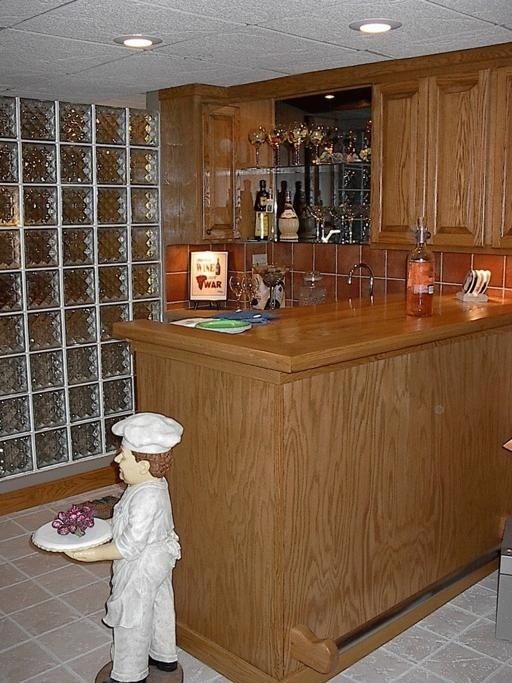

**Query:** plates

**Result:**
xmin=461 ymin=269 xmax=492 ymax=297
xmin=195 ymin=318 xmax=252 ymax=333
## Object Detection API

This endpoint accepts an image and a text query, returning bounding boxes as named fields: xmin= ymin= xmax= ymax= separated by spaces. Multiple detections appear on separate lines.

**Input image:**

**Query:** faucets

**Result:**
xmin=348 ymin=260 xmax=375 ymax=296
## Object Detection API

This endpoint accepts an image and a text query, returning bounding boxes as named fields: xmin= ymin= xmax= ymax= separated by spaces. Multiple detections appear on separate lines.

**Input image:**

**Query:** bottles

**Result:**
xmin=277 ymin=178 xmax=291 ymax=240
xmin=254 ymin=179 xmax=276 ymax=240
xmin=297 ymin=268 xmax=328 ymax=306
xmin=293 ymin=179 xmax=306 ymax=215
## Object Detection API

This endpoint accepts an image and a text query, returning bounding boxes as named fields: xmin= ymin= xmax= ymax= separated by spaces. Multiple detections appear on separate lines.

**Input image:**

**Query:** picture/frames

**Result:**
xmin=188 ymin=249 xmax=229 ymax=302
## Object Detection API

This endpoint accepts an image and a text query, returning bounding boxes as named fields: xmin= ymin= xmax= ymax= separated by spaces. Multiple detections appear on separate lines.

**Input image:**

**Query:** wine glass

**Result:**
xmin=285 ymin=119 xmax=308 ymax=166
xmin=307 ymin=204 xmax=372 ymax=244
xmin=308 ymin=124 xmax=324 ymax=165
xmin=265 ymin=121 xmax=287 ymax=167
xmin=248 ymin=122 xmax=266 ymax=168
xmin=242 ymin=275 xmax=259 ymax=313
xmin=230 ymin=275 xmax=244 ymax=312
xmin=254 ymin=262 xmax=290 ymax=313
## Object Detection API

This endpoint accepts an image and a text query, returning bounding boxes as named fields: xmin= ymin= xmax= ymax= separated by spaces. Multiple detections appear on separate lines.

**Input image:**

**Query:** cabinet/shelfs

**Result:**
xmin=245 ymin=63 xmax=377 ymax=247
xmin=374 ymin=43 xmax=512 ymax=254
xmin=155 ymin=82 xmax=244 ymax=244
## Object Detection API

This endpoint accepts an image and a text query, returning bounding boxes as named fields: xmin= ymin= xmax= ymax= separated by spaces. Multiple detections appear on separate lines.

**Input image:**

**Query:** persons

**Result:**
xmin=60 ymin=413 xmax=182 ymax=682
